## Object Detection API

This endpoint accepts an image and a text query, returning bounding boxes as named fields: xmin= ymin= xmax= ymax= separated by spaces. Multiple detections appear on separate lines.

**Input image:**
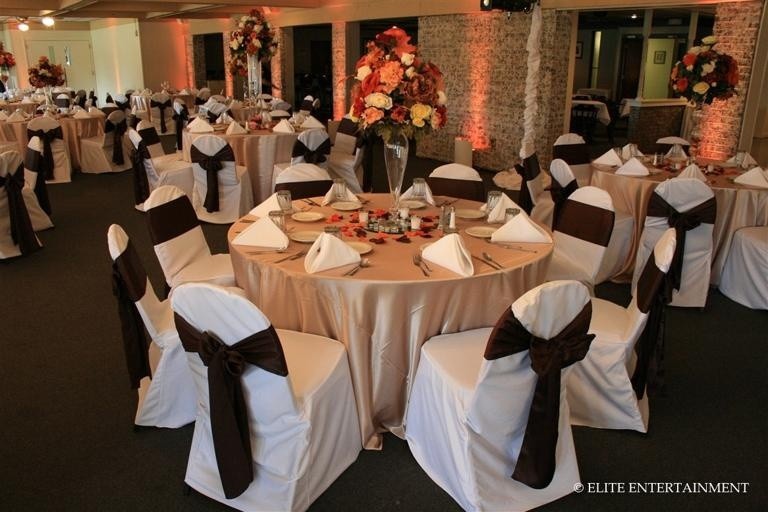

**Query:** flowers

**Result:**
xmin=336 ymin=27 xmax=448 ymax=139
xmin=670 ymin=35 xmax=739 ymax=107
xmin=228 ymin=8 xmax=278 ymax=78
xmin=27 ymin=56 xmax=65 ymax=88
xmin=0 ymin=41 xmax=15 ymax=68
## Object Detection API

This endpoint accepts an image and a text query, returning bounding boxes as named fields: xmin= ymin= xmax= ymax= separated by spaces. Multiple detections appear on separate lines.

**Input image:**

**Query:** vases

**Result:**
xmin=43 ymin=84 xmax=52 ymax=106
xmin=0 ymin=64 xmax=10 ymax=90
xmin=246 ymin=52 xmax=262 ymax=101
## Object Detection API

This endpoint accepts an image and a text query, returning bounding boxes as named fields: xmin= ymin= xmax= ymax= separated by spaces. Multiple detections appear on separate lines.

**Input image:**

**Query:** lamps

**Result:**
xmin=42 ymin=15 xmax=55 ymax=27
xmin=14 ymin=16 xmax=30 ymax=32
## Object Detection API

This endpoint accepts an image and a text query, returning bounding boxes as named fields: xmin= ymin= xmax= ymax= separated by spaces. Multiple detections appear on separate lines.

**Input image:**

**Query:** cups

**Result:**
xmin=196 ymin=106 xmax=304 ymax=133
xmin=613 ymin=142 xmax=759 ymax=182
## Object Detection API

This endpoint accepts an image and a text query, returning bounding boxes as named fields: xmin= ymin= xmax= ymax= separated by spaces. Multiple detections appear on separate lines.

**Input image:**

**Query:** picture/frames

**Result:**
xmin=575 ymin=41 xmax=584 ymax=59
xmin=654 ymin=50 xmax=666 ymax=63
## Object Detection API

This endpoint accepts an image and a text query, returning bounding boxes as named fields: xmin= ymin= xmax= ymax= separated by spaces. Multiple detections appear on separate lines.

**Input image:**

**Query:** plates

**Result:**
xmin=234 ymin=186 xmax=541 ymax=282
